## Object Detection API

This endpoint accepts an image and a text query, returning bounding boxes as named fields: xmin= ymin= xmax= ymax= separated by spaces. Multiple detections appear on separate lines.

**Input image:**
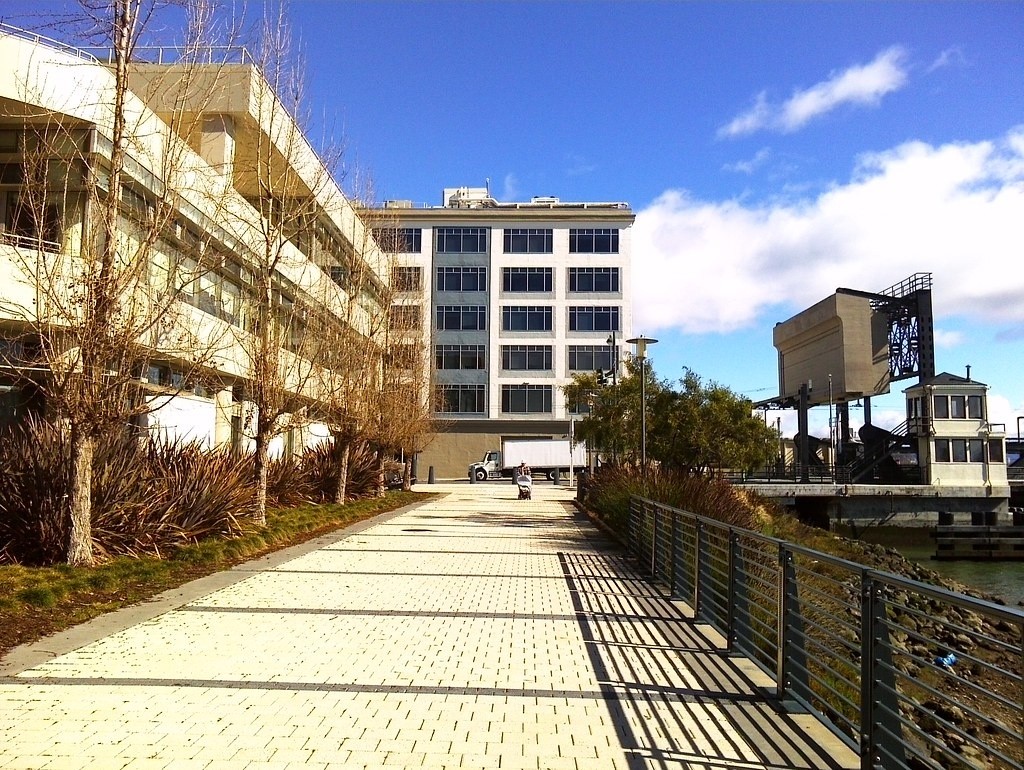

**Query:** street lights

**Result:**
xmin=607 ymin=330 xmax=617 ymax=384
xmin=627 ymin=334 xmax=659 ymax=484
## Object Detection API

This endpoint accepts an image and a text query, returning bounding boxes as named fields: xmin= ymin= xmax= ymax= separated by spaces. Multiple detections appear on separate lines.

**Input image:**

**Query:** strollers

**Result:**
xmin=517 ymin=475 xmax=532 ymax=500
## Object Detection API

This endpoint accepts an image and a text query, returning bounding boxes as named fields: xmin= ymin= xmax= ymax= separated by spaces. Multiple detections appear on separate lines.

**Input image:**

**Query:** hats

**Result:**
xmin=521 ymin=460 xmax=526 ymax=463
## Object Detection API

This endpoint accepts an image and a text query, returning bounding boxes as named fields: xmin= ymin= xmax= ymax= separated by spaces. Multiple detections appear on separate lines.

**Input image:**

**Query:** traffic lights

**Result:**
xmin=597 ymin=369 xmax=602 ymax=384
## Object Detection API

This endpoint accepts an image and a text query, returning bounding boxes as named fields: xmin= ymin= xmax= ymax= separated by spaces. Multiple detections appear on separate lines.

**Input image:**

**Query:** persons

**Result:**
xmin=517 ymin=460 xmax=531 ymax=499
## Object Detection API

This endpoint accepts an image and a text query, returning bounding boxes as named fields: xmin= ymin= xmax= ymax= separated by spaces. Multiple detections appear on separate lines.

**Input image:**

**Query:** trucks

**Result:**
xmin=468 ymin=439 xmax=586 ymax=481
